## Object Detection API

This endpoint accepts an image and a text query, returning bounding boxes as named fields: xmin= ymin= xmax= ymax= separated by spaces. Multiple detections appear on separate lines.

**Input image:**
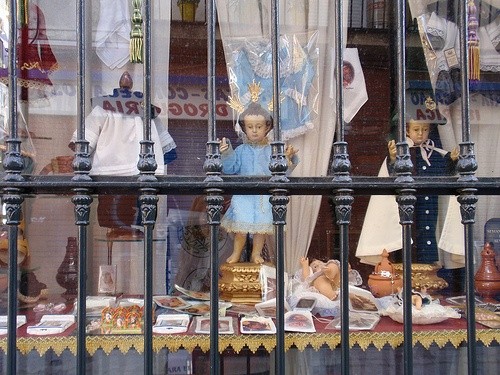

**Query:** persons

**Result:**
xmin=216 ymin=101 xmax=299 ymax=264
xmin=68 ymin=71 xmax=178 ymax=239
xmin=354 ymin=107 xmax=478 ymax=270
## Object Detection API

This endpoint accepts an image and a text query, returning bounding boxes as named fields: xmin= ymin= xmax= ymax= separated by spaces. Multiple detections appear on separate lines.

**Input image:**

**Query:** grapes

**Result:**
xmin=19 ymin=269 xmax=47 ymax=297
